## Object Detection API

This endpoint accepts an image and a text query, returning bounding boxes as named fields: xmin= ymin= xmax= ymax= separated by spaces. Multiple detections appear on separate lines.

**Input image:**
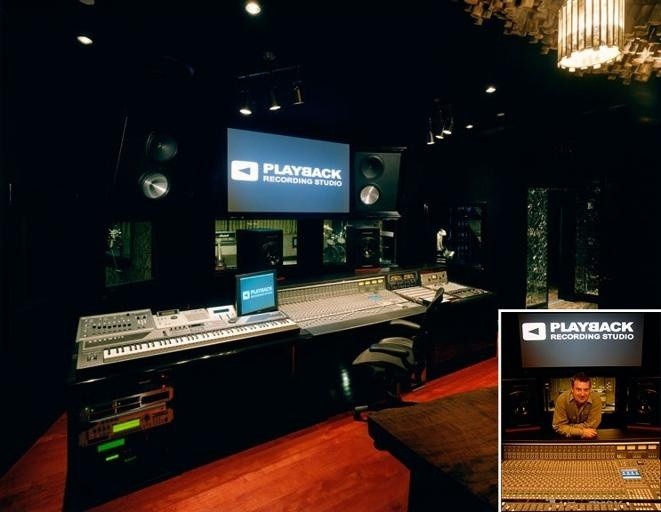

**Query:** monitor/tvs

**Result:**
xmin=232 ymin=269 xmax=279 ymax=316
xmin=222 ymin=123 xmax=360 ymax=220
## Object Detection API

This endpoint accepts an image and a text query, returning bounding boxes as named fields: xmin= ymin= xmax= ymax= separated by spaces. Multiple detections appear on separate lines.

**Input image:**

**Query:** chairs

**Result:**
xmin=626 ymin=377 xmax=661 ymax=432
xmin=352 ymin=288 xmax=444 ymax=419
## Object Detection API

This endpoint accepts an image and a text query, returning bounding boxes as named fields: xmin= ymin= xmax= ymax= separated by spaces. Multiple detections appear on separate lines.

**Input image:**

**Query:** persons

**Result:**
xmin=551 ymin=372 xmax=604 ymax=440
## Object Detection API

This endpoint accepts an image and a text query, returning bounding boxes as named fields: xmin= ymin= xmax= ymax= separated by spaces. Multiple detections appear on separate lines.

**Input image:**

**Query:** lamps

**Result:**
xmin=555 ymin=0 xmax=625 ymax=72
xmin=237 ymin=65 xmax=303 ymax=116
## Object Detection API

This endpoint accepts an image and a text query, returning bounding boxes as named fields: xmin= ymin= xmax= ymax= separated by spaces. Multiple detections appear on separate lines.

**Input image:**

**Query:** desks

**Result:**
xmin=368 ymin=386 xmax=497 ymax=512
xmin=502 ymin=378 xmax=554 ymax=432
xmin=62 ymin=292 xmax=496 ymax=512
xmin=502 ymin=428 xmax=661 ymax=442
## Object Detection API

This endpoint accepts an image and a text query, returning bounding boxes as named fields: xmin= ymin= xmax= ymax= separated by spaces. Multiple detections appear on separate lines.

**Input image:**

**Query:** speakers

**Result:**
xmin=354 ymin=150 xmax=403 ymax=211
xmin=107 ymin=112 xmax=204 ymax=221
xmin=346 ymin=226 xmax=380 ymax=268
xmin=236 ymin=228 xmax=283 ymax=272
